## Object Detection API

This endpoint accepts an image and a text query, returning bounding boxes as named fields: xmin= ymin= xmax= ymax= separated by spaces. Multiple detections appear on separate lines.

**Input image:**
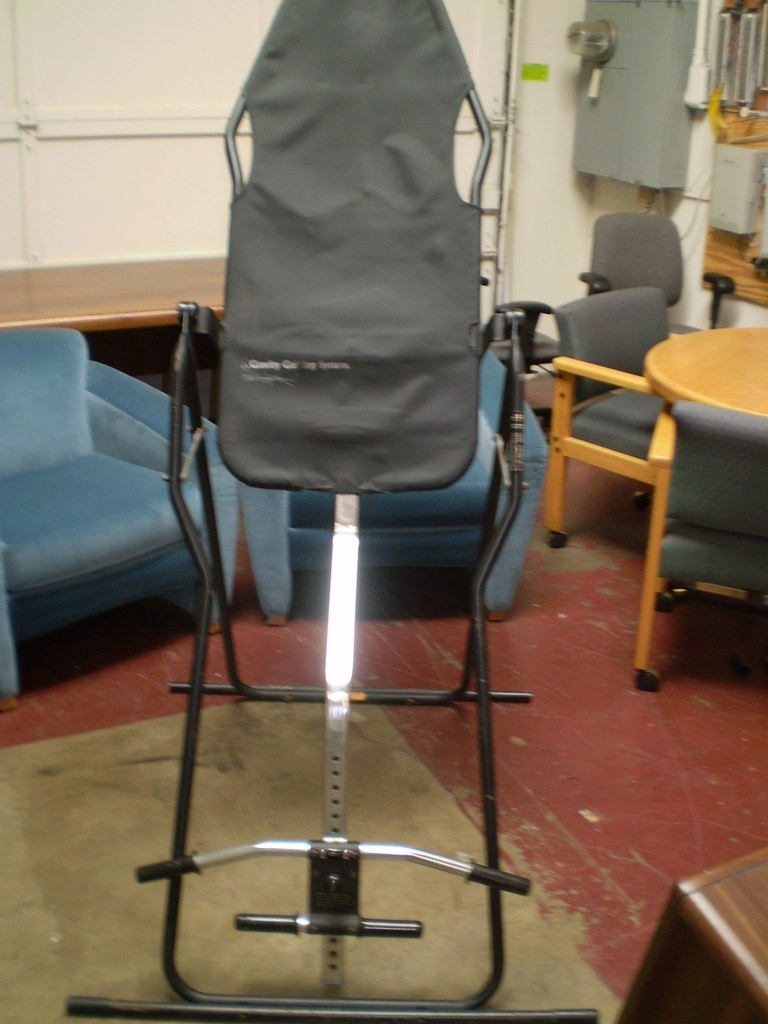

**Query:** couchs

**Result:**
xmin=239 ymin=347 xmax=552 ymax=623
xmin=2 ymin=325 xmax=243 ymax=713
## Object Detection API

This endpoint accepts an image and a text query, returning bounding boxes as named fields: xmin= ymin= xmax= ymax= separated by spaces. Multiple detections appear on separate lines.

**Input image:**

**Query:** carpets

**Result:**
xmin=0 ymin=678 xmax=623 ymax=1024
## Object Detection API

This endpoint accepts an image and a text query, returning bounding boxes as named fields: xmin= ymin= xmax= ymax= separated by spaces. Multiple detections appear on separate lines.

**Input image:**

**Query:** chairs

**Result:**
xmin=634 ymin=399 xmax=768 ymax=690
xmin=546 ymin=285 xmax=662 ymax=550
xmin=580 ymin=211 xmax=735 ymax=343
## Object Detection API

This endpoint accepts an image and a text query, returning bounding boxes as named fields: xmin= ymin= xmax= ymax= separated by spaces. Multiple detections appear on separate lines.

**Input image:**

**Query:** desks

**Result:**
xmin=0 ymin=256 xmax=227 ymax=332
xmin=643 ymin=328 xmax=768 ymax=420
